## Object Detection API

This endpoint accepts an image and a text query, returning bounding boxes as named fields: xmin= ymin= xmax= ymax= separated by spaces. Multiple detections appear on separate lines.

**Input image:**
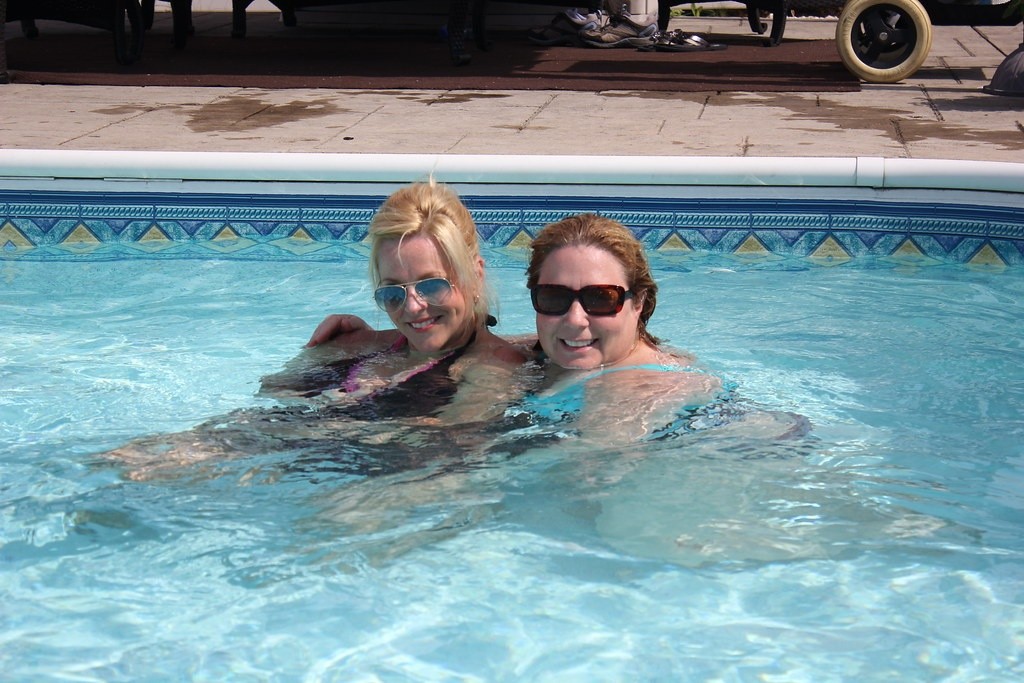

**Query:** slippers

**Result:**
xmin=637 ymin=29 xmax=727 ymax=52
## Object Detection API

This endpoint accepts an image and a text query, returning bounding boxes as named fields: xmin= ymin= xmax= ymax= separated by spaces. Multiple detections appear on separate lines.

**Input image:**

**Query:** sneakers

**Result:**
xmin=578 ymin=12 xmax=660 ymax=47
xmin=528 ymin=8 xmax=603 ymax=47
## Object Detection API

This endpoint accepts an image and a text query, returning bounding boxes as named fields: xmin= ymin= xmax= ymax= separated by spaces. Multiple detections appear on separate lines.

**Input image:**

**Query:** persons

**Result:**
xmin=301 ymin=210 xmax=983 ymax=564
xmin=86 ymin=175 xmax=534 ymax=502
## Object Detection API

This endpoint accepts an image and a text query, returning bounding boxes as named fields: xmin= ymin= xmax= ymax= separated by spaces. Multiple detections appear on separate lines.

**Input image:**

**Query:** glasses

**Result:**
xmin=531 ymin=284 xmax=638 ymax=317
xmin=372 ymin=278 xmax=459 ymax=314
